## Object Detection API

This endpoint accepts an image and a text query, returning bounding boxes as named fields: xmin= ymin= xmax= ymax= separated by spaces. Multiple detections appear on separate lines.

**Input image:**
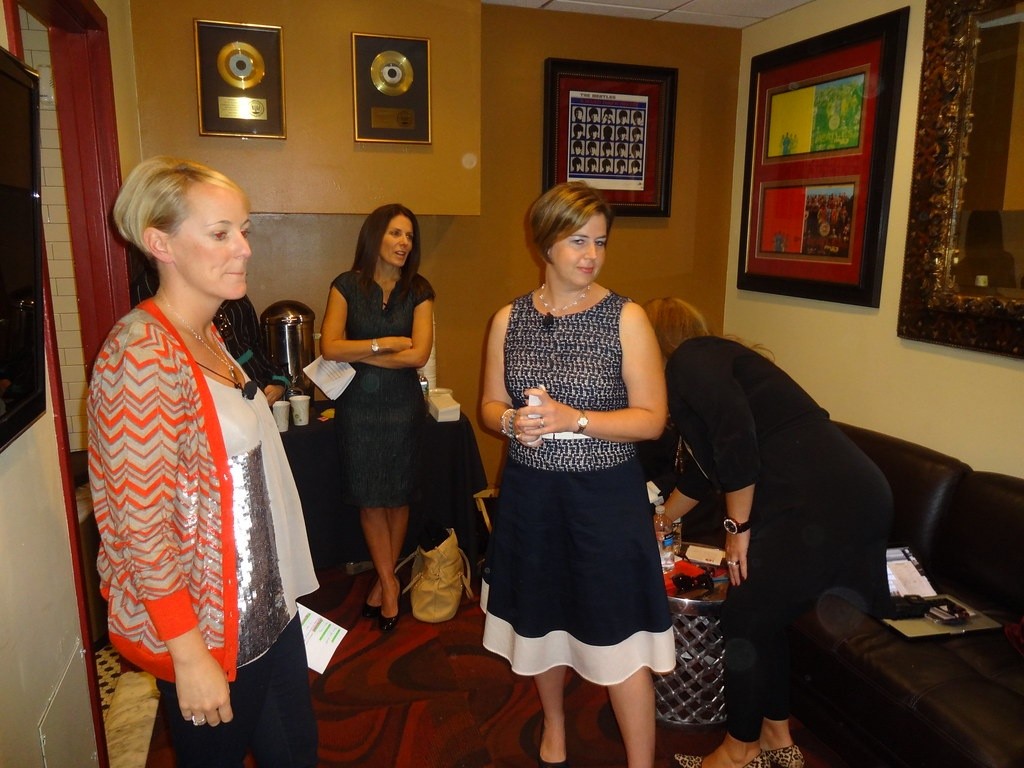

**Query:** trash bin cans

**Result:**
xmin=70 ymin=450 xmax=109 ymax=651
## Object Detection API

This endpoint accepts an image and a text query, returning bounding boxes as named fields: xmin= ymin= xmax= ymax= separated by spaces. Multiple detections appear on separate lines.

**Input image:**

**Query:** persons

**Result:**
xmin=319 ymin=204 xmax=436 ymax=634
xmin=643 ymin=298 xmax=895 ymax=768
xmin=86 ymin=155 xmax=319 ymax=767
xmin=480 ymin=182 xmax=675 ymax=768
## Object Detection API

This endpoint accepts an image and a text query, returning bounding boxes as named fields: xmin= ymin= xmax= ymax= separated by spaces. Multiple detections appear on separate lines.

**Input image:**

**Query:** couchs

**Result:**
xmin=669 ymin=416 xmax=1024 ymax=768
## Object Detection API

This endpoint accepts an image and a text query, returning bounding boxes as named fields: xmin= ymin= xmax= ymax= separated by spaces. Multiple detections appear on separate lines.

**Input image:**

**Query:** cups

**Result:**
xmin=273 ymin=401 xmax=290 ymax=432
xmin=289 ymin=395 xmax=310 ymax=426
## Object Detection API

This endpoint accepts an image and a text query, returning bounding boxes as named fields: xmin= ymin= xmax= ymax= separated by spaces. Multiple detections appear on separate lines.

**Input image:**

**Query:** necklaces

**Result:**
xmin=156 ymin=290 xmax=236 ymax=378
xmin=539 ymin=283 xmax=590 ymax=315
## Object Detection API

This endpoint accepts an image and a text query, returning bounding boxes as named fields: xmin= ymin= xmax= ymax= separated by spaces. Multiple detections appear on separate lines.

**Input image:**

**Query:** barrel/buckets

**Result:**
xmin=260 ymin=300 xmax=315 ymax=401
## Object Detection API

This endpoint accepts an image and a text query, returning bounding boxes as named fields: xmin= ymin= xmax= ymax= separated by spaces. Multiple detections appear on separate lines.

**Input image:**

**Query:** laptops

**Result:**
xmin=880 ymin=547 xmax=1002 ymax=640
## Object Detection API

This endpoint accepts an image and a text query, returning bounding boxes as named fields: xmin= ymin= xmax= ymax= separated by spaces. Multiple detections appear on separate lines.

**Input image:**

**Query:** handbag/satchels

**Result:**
xmin=663 ymin=560 xmax=714 ymax=598
xmin=393 ymin=527 xmax=473 ymax=623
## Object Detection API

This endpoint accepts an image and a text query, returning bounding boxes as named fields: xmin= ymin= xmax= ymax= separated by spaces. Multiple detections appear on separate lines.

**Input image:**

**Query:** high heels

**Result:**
xmin=361 ymin=600 xmax=381 ymax=618
xmin=379 ymin=573 xmax=404 ymax=632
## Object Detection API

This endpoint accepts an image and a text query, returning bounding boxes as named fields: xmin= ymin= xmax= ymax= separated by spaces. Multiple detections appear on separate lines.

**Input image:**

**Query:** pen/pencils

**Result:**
xmin=713 ymin=577 xmax=728 ymax=582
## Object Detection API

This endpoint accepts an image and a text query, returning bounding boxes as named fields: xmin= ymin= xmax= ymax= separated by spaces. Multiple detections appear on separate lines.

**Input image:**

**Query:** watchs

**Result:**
xmin=573 ymin=410 xmax=589 ymax=433
xmin=507 ymin=410 xmax=515 ymax=439
xmin=723 ymin=516 xmax=751 ymax=534
xmin=370 ymin=338 xmax=381 ymax=355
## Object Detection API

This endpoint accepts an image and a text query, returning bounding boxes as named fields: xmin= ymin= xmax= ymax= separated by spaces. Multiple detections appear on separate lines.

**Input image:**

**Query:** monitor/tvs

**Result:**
xmin=0 ymin=43 xmax=47 ymax=454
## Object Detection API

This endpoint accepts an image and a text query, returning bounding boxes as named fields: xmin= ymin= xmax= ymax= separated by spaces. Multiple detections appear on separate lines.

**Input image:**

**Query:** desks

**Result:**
xmin=273 ymin=397 xmax=491 ymax=566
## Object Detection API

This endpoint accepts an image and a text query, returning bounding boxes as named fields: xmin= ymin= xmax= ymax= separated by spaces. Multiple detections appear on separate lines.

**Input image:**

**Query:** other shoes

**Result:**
xmin=536 ymin=750 xmax=570 ymax=768
xmin=345 ymin=559 xmax=374 ymax=575
xmin=674 ymin=749 xmax=771 ymax=768
xmin=765 ymin=744 xmax=807 ymax=768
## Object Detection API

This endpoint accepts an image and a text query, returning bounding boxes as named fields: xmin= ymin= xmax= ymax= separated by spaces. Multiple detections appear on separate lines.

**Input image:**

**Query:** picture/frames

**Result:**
xmin=350 ymin=31 xmax=432 ymax=145
xmin=193 ymin=18 xmax=287 ymax=140
xmin=541 ymin=56 xmax=678 ymax=218
xmin=734 ymin=5 xmax=910 ymax=308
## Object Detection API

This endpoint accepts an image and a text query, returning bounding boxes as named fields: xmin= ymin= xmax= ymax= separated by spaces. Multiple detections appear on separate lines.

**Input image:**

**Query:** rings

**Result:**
xmin=540 ymin=417 xmax=544 ymax=427
xmin=727 ymin=561 xmax=738 ymax=566
xmin=191 ymin=715 xmax=205 ymax=726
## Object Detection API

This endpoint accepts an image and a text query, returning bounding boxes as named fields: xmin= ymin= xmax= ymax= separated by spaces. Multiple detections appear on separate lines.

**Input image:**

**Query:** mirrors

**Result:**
xmin=897 ymin=0 xmax=1024 ymax=363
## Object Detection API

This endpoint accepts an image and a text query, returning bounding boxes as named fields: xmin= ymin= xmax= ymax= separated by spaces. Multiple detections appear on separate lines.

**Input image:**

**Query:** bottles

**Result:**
xmin=418 ymin=370 xmax=429 ymax=416
xmin=672 ymin=516 xmax=682 ymax=554
xmin=653 ymin=506 xmax=675 ymax=575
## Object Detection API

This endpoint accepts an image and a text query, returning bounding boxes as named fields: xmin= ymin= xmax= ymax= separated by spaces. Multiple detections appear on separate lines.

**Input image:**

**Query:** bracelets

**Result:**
xmin=501 ymin=408 xmax=514 ymax=436
